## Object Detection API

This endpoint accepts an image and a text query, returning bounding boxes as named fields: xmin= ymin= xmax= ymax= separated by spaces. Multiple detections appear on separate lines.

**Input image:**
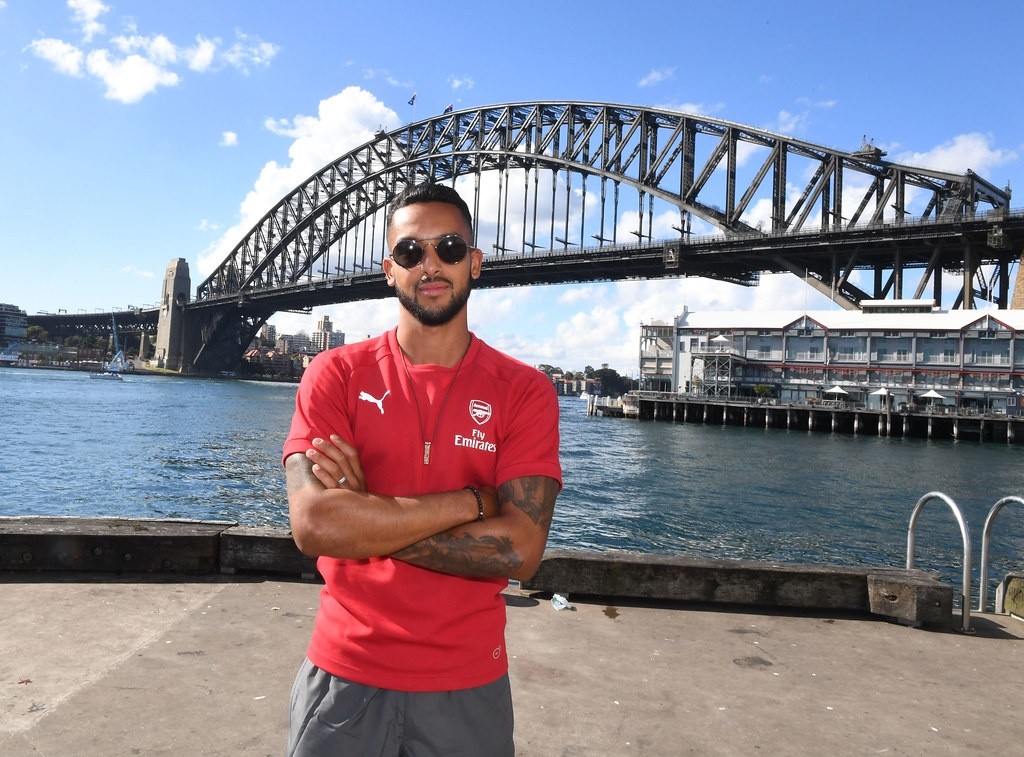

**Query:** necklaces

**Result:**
xmin=399 ymin=335 xmax=472 ymax=465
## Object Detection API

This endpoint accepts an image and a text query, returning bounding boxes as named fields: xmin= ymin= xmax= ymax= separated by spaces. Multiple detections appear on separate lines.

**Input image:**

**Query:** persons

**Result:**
xmin=281 ymin=183 xmax=563 ymax=757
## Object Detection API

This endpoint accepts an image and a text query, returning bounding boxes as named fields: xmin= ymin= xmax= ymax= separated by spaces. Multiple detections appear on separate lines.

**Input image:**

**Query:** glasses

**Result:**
xmin=389 ymin=235 xmax=475 ymax=268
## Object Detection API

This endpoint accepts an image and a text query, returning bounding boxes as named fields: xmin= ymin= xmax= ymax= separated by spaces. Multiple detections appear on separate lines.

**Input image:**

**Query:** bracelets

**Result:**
xmin=463 ymin=485 xmax=484 ymax=522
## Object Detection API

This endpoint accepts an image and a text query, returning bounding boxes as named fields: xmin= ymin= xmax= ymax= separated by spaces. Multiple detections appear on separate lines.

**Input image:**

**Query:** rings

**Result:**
xmin=338 ymin=476 xmax=347 ymax=485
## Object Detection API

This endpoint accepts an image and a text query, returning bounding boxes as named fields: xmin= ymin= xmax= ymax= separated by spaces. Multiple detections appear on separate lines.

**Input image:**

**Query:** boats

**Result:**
xmin=90 ymin=372 xmax=122 ymax=380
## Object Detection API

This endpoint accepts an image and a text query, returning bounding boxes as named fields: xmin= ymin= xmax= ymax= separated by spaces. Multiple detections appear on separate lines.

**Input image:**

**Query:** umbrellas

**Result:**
xmin=920 ymin=389 xmax=946 ymax=405
xmin=870 ymin=388 xmax=894 ymax=406
xmin=825 ymin=386 xmax=849 ymax=400
